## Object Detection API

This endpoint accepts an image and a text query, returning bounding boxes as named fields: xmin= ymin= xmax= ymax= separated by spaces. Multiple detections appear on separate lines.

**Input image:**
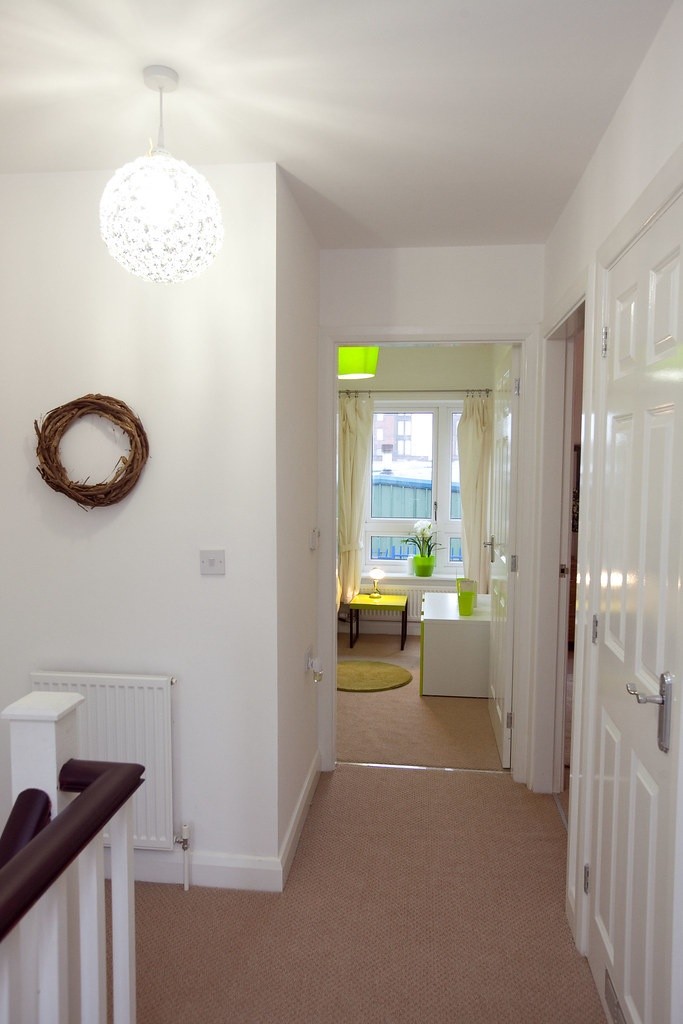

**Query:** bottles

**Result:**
xmin=407 ymin=555 xmax=414 ymax=574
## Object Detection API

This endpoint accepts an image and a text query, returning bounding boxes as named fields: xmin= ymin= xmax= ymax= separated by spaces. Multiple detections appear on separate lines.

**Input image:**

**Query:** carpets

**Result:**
xmin=336 ymin=658 xmax=412 ymax=693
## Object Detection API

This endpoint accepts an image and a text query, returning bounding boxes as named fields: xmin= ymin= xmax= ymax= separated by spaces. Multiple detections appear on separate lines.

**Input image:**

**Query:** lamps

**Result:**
xmin=369 ymin=568 xmax=385 ymax=598
xmin=97 ymin=66 xmax=222 ymax=284
xmin=337 ymin=346 xmax=379 ymax=380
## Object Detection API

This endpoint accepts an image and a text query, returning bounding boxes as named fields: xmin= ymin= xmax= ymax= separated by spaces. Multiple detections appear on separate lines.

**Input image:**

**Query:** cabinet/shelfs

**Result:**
xmin=417 ymin=590 xmax=492 ymax=701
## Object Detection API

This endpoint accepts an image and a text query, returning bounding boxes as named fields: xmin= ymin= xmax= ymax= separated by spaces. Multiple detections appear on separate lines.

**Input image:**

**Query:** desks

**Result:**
xmin=348 ymin=595 xmax=410 ymax=649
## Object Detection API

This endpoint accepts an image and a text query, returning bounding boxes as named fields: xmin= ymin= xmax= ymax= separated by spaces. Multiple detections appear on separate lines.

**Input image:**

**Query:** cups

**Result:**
xmin=459 ymin=581 xmax=474 ymax=616
xmin=457 ymin=578 xmax=469 ymax=591
xmin=474 ymin=582 xmax=477 ymax=608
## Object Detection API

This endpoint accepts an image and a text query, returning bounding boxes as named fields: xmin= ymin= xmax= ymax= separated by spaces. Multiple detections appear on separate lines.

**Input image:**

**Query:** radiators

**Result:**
xmin=28 ymin=671 xmax=190 ymax=853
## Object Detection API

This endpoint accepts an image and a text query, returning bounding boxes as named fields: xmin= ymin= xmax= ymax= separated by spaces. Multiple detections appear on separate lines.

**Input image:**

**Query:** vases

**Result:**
xmin=413 ymin=554 xmax=436 ymax=577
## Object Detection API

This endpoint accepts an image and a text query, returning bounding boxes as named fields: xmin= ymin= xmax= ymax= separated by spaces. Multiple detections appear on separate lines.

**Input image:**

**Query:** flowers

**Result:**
xmin=401 ymin=519 xmax=446 ymax=557
